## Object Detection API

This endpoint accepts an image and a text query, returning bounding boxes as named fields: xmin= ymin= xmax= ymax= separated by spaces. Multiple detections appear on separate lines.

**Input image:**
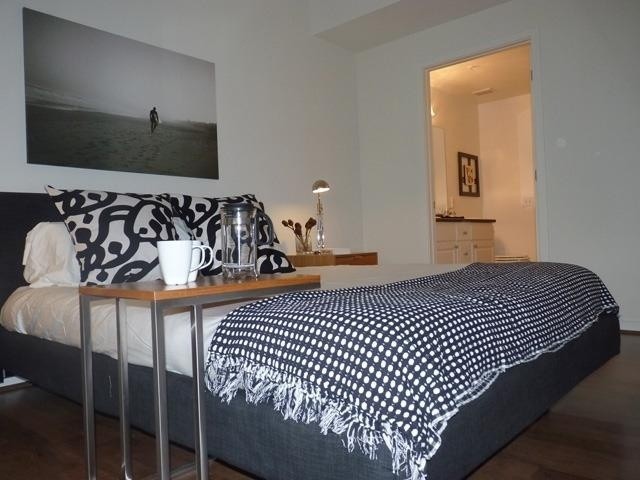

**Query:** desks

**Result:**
xmin=73 ymin=272 xmax=327 ymax=474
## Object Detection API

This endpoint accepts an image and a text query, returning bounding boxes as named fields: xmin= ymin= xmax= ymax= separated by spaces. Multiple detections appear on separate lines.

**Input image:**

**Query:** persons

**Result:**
xmin=150 ymin=107 xmax=159 ymax=133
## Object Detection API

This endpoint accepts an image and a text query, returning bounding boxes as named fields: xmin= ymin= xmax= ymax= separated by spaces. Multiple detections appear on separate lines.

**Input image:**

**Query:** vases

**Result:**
xmin=295 ymin=236 xmax=314 ymax=254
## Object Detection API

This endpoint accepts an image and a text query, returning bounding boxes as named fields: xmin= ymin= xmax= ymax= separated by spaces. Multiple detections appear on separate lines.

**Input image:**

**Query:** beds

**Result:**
xmin=1 ymin=189 xmax=624 ymax=480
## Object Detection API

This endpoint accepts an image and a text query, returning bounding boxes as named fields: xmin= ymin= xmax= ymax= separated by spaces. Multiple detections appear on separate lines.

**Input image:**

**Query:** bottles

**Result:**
xmin=220 ymin=203 xmax=259 ymax=280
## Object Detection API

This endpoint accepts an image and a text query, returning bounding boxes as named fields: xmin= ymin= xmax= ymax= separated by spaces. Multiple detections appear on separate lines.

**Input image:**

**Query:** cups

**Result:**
xmin=156 ymin=239 xmax=214 ymax=286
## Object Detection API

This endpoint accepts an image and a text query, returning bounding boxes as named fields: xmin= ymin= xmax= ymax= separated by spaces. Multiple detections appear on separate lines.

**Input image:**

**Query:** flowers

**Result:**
xmin=281 ymin=216 xmax=318 ymax=250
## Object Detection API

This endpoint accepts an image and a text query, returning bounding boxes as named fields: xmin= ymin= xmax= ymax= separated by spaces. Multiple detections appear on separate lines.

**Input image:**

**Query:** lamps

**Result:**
xmin=310 ymin=180 xmax=330 ymax=249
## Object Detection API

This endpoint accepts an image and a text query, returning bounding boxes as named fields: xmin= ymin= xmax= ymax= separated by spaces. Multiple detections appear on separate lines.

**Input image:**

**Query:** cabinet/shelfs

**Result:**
xmin=435 ymin=221 xmax=495 ymax=267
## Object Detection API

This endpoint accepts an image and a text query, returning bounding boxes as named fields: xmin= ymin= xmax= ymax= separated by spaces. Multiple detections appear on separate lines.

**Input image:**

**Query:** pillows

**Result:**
xmin=21 ymin=185 xmax=297 ymax=291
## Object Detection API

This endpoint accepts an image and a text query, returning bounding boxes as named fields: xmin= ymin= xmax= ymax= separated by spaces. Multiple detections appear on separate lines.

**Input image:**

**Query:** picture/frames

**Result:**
xmin=457 ymin=149 xmax=482 ymax=199
